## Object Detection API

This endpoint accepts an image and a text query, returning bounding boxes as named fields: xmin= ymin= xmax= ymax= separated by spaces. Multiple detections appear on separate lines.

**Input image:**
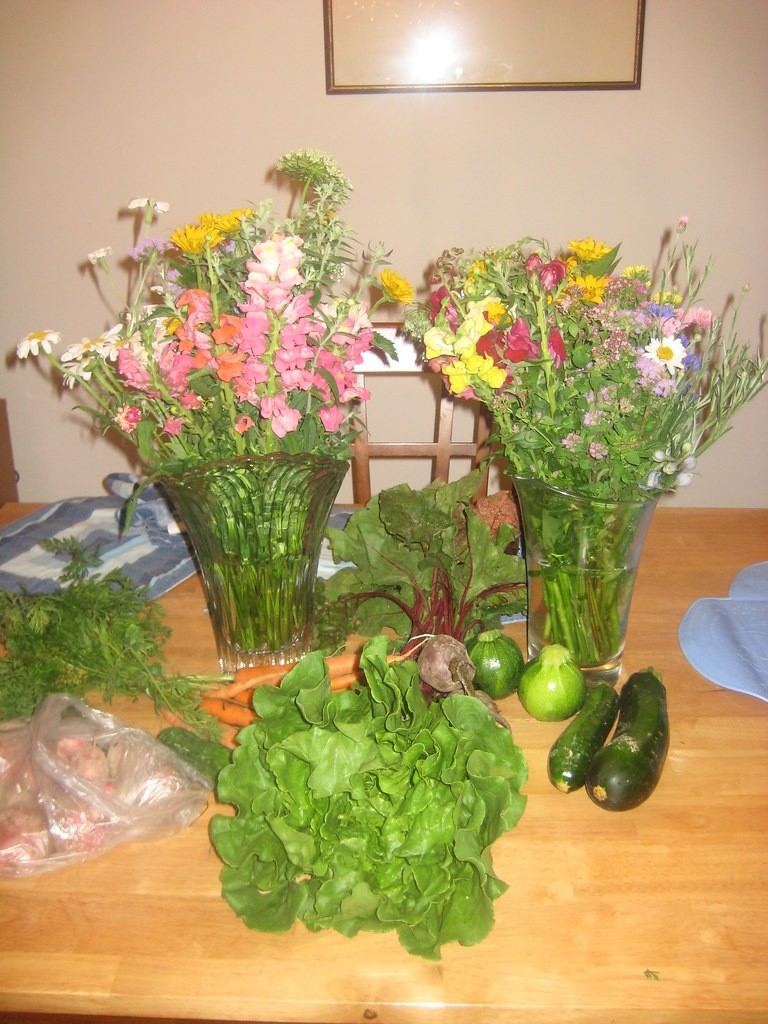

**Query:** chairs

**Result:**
xmin=349 ymin=323 xmax=492 ymax=505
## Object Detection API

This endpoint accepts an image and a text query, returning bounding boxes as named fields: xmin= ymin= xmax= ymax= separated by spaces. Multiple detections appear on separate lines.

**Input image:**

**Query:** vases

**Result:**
xmin=512 ymin=476 xmax=657 ymax=688
xmin=156 ymin=451 xmax=351 ymax=675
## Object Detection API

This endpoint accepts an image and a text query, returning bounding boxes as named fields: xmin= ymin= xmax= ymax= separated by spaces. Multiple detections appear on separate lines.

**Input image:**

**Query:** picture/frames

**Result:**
xmin=323 ymin=0 xmax=646 ymax=96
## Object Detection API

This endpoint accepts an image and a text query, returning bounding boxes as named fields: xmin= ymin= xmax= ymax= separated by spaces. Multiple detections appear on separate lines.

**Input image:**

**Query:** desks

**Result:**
xmin=0 ymin=502 xmax=768 ymax=1024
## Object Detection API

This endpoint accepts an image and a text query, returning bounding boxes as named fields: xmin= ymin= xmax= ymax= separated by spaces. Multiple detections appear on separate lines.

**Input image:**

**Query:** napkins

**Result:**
xmin=0 ymin=496 xmax=200 ymax=603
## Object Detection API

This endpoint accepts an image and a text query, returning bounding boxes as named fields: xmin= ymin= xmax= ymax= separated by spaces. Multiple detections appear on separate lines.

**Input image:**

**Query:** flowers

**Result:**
xmin=404 ymin=218 xmax=768 ymax=501
xmin=14 ymin=147 xmax=416 ymax=541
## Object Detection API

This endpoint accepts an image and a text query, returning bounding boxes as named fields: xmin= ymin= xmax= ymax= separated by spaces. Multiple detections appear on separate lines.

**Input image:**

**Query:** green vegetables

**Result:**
xmin=315 ymin=467 xmax=528 ymax=692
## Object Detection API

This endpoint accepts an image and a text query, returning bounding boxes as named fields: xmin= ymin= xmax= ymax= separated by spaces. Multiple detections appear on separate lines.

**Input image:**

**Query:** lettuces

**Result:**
xmin=208 ymin=632 xmax=529 ymax=962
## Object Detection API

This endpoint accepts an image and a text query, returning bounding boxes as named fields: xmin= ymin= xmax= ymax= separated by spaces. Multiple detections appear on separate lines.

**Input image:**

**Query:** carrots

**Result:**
xmin=160 ymin=637 xmax=430 ymax=751
